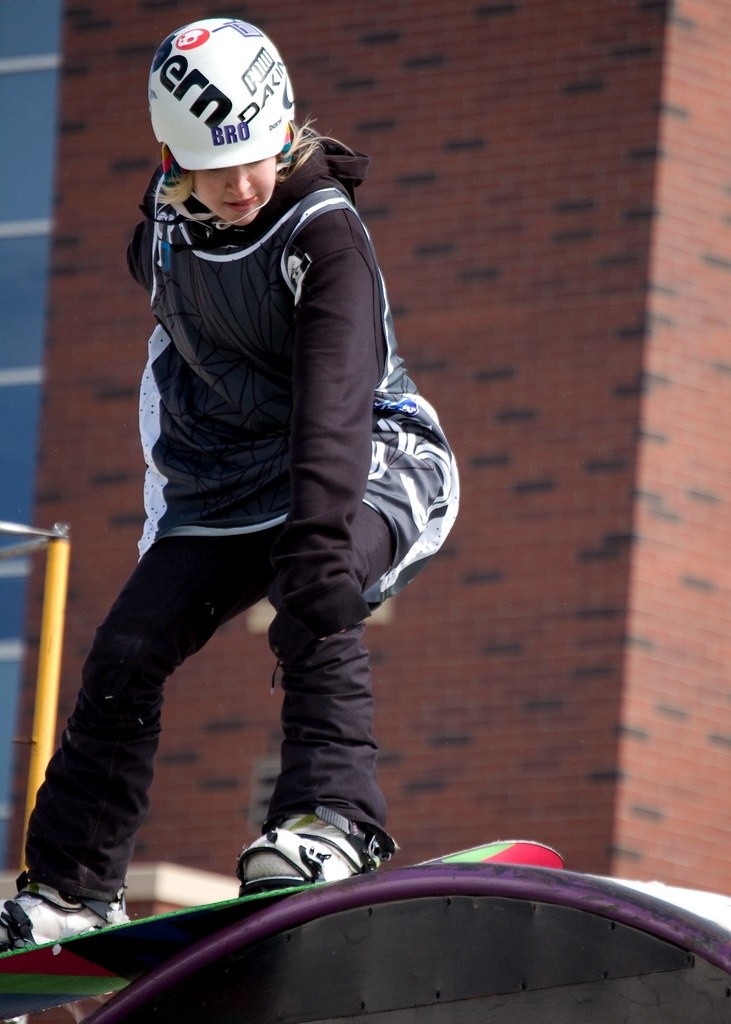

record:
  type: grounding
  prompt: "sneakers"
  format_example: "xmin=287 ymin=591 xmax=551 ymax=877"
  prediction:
xmin=244 ymin=806 xmax=385 ymax=879
xmin=0 ymin=879 xmax=130 ymax=951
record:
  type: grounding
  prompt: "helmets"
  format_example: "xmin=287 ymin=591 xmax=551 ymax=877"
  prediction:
xmin=147 ymin=17 xmax=295 ymax=172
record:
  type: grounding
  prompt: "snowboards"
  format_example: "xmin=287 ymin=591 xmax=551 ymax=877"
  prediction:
xmin=1 ymin=839 xmax=562 ymax=1019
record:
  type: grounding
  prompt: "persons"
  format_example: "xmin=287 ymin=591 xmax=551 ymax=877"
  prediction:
xmin=0 ymin=15 xmax=462 ymax=953
